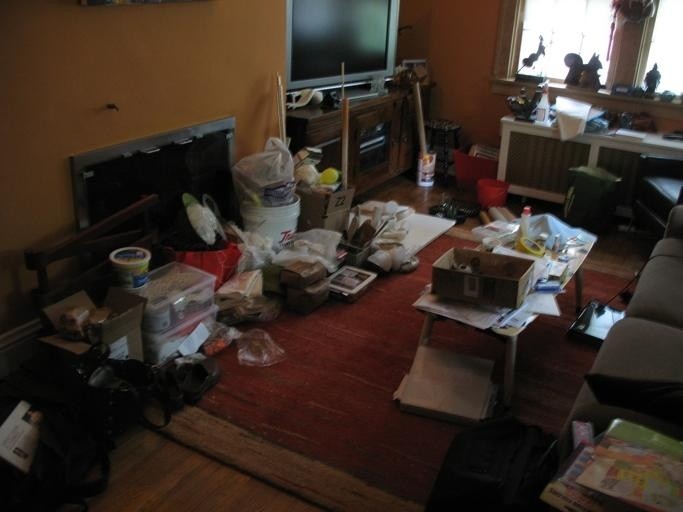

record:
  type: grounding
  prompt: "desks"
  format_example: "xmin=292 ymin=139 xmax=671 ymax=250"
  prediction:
xmin=412 ymin=212 xmax=598 ymax=407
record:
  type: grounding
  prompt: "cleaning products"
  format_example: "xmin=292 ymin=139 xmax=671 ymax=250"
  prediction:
xmin=535 ymin=80 xmax=550 ymax=126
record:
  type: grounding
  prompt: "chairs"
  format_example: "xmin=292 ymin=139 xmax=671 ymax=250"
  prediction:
xmin=630 ymin=131 xmax=682 ymax=227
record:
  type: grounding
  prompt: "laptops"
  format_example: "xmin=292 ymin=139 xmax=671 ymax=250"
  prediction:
xmin=568 ymin=299 xmax=625 ymax=344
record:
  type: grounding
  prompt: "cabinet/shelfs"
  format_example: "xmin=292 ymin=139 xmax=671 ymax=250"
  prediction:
xmin=404 ymin=82 xmax=438 ymax=173
xmin=497 ymin=110 xmax=683 ymax=220
xmin=288 ymin=84 xmax=409 ymax=198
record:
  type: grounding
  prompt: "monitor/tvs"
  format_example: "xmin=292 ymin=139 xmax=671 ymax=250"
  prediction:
xmin=286 ymin=0 xmax=402 ymax=90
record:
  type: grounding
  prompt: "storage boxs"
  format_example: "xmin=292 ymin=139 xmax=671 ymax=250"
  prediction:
xmin=295 ymin=186 xmax=356 ymax=235
xmin=117 ymin=261 xmax=218 ymax=336
xmin=140 ymin=304 xmax=220 ymax=366
xmin=431 ymin=245 xmax=534 ymax=309
xmin=38 ymin=286 xmax=150 ymax=364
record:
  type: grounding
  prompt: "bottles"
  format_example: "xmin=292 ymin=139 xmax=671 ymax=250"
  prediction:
xmin=536 ymin=87 xmax=549 ymax=123
xmin=521 ymin=207 xmax=531 ymax=238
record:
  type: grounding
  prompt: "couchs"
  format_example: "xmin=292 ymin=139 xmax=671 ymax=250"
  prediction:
xmin=558 ymin=206 xmax=682 ymax=464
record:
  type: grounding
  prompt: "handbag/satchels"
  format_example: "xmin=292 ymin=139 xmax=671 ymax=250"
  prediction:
xmin=93 ymin=358 xmax=153 ymax=441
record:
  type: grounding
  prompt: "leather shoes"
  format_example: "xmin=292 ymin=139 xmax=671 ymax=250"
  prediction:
xmin=169 ymin=357 xmax=220 ymax=409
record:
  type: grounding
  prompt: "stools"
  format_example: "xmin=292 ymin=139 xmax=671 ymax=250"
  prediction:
xmin=424 ymin=117 xmax=461 ymax=178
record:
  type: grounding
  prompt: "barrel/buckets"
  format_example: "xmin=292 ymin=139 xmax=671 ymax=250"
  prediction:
xmin=476 ymin=178 xmax=510 ymax=209
xmin=109 ymin=245 xmax=152 ymax=289
xmin=241 ymin=194 xmax=302 ymax=249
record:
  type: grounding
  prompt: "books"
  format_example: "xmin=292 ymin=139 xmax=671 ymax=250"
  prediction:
xmin=326 ymin=264 xmax=377 ymax=297
xmin=615 ymin=128 xmax=649 ymax=142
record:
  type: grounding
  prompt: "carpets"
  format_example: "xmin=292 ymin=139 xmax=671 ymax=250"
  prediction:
xmin=138 ymin=225 xmax=637 ymax=512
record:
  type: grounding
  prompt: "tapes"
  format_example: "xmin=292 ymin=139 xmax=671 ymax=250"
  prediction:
xmin=517 ymin=237 xmax=545 ymax=258
